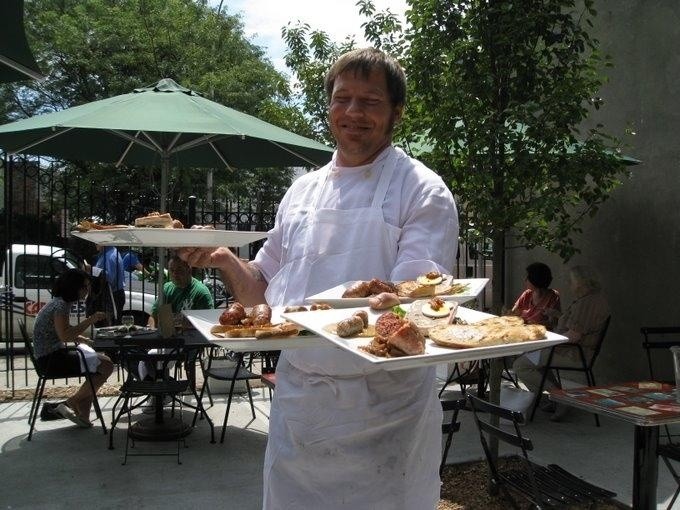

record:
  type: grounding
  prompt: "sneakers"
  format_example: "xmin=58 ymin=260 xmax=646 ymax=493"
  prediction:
xmin=541 ymin=401 xmax=571 ymax=420
xmin=143 ymin=393 xmax=174 ymax=414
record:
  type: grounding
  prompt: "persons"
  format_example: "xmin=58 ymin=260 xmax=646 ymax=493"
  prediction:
xmin=122 ymin=246 xmax=153 ymax=283
xmin=500 ymin=261 xmax=567 ymax=334
xmin=135 ymin=256 xmax=214 ymax=413
xmin=84 ymin=242 xmax=126 ymax=328
xmin=175 ymin=46 xmax=463 ymax=509
xmin=31 ymin=266 xmax=116 ymax=430
xmin=511 ymin=263 xmax=613 ymax=427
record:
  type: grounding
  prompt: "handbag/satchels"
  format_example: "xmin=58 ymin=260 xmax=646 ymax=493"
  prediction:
xmin=42 ymin=402 xmax=69 ymax=420
xmin=499 ymin=385 xmax=536 ymax=426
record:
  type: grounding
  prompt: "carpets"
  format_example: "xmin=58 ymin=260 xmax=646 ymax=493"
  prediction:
xmin=438 ymin=454 xmax=636 ymax=510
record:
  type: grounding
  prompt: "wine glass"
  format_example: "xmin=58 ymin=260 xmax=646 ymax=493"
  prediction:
xmin=121 ymin=316 xmax=134 ymax=335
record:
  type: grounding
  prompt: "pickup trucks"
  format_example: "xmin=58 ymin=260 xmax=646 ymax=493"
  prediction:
xmin=0 ymin=243 xmax=156 ymax=343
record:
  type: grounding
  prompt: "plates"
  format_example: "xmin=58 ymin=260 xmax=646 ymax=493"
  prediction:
xmin=179 ymin=304 xmax=334 ymax=352
xmin=303 ymin=276 xmax=489 ymax=308
xmin=278 ymin=301 xmax=572 ymax=373
xmin=94 ymin=325 xmax=160 ymax=338
xmin=70 ymin=226 xmax=275 ymax=248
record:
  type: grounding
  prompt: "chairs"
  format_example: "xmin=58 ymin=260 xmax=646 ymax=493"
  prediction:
xmin=17 ymin=309 xmax=263 ymax=470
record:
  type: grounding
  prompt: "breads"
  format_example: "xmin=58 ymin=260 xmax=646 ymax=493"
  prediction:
xmin=135 ymin=214 xmax=173 ymax=227
xmin=404 ymin=298 xmax=459 ymax=338
xmin=428 ymin=315 xmax=546 ymax=349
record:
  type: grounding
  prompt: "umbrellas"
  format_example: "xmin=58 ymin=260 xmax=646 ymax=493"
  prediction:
xmin=0 ymin=73 xmax=338 ymax=310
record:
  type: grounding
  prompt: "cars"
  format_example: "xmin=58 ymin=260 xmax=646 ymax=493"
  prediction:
xmin=469 ymin=237 xmax=494 ymax=260
xmin=131 ymin=255 xmax=227 ymax=309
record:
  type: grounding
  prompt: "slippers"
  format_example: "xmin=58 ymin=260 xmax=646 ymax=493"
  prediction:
xmin=57 ymin=404 xmax=92 ymax=427
